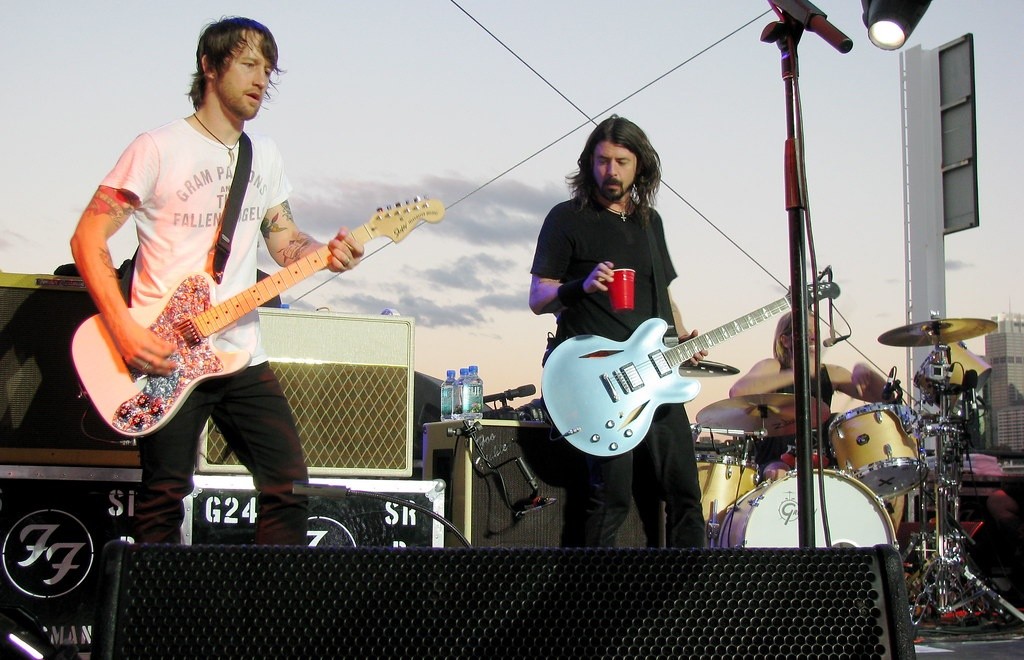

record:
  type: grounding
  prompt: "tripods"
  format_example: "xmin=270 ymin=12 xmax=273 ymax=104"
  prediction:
xmin=870 ymin=344 xmax=1024 ymax=625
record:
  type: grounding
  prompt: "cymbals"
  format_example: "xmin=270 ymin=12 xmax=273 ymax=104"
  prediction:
xmin=877 ymin=317 xmax=998 ymax=349
xmin=695 ymin=390 xmax=831 ymax=440
xmin=680 ymin=360 xmax=742 ymax=378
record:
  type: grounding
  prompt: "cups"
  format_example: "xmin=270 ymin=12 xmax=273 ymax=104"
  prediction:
xmin=607 ymin=269 xmax=635 ymax=313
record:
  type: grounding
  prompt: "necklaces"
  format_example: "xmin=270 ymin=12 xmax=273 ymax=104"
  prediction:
xmin=193 ymin=113 xmax=239 ymax=167
xmin=603 ymin=198 xmax=632 ymax=221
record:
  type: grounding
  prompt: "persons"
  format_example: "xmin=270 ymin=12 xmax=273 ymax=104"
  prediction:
xmin=529 ymin=114 xmax=708 ymax=547
xmin=70 ymin=18 xmax=364 ymax=544
xmin=729 ymin=311 xmax=896 ymax=483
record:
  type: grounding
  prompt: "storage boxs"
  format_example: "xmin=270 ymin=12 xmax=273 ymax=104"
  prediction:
xmin=1 ymin=466 xmax=446 ymax=660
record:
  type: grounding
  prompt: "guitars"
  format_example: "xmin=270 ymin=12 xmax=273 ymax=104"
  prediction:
xmin=542 ymin=276 xmax=840 ymax=457
xmin=69 ymin=193 xmax=445 ymax=436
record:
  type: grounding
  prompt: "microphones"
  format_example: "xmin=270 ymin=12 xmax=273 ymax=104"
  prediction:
xmin=880 ymin=365 xmax=900 ymax=401
xmin=823 ymin=335 xmax=848 ymax=348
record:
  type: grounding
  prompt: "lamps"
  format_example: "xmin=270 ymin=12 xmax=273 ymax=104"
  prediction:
xmin=860 ymin=0 xmax=934 ymax=50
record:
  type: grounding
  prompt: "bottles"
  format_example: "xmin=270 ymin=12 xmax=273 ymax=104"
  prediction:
xmin=441 ymin=370 xmax=457 ymax=422
xmin=451 ymin=368 xmax=469 ymax=420
xmin=462 ymin=366 xmax=483 ymax=419
xmin=281 ymin=304 xmax=289 ymax=308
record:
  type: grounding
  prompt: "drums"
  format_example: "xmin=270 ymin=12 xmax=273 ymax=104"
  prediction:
xmin=913 ymin=342 xmax=996 ymax=412
xmin=695 ymin=454 xmax=900 ymax=551
xmin=825 ymin=402 xmax=930 ymax=502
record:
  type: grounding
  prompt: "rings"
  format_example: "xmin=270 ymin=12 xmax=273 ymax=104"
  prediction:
xmin=140 ymin=363 xmax=151 ymax=374
xmin=344 ymin=258 xmax=350 ymax=266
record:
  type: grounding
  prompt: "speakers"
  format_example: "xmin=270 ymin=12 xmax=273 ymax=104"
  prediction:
xmin=195 ymin=307 xmax=414 ymax=478
xmin=88 ymin=536 xmax=919 ymax=660
xmin=5 ymin=468 xmax=183 ymax=651
xmin=422 ymin=421 xmax=667 ymax=548
xmin=0 ymin=271 xmax=141 ymax=467
xmin=182 ymin=480 xmax=447 ymax=548
